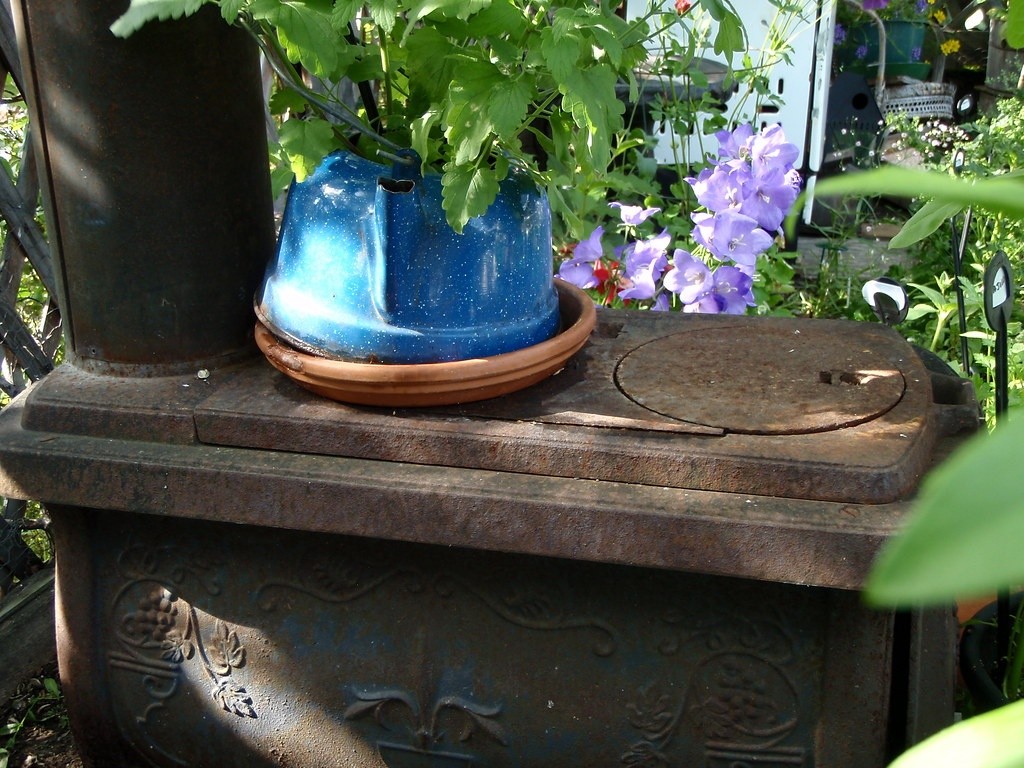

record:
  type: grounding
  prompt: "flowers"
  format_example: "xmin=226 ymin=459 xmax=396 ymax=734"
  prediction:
xmin=556 ymin=122 xmax=804 ymax=316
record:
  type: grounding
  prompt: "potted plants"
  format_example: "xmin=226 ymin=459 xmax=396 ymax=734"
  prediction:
xmin=110 ymin=1 xmax=750 ymax=397
xmin=837 ymin=1 xmax=932 ymax=82
